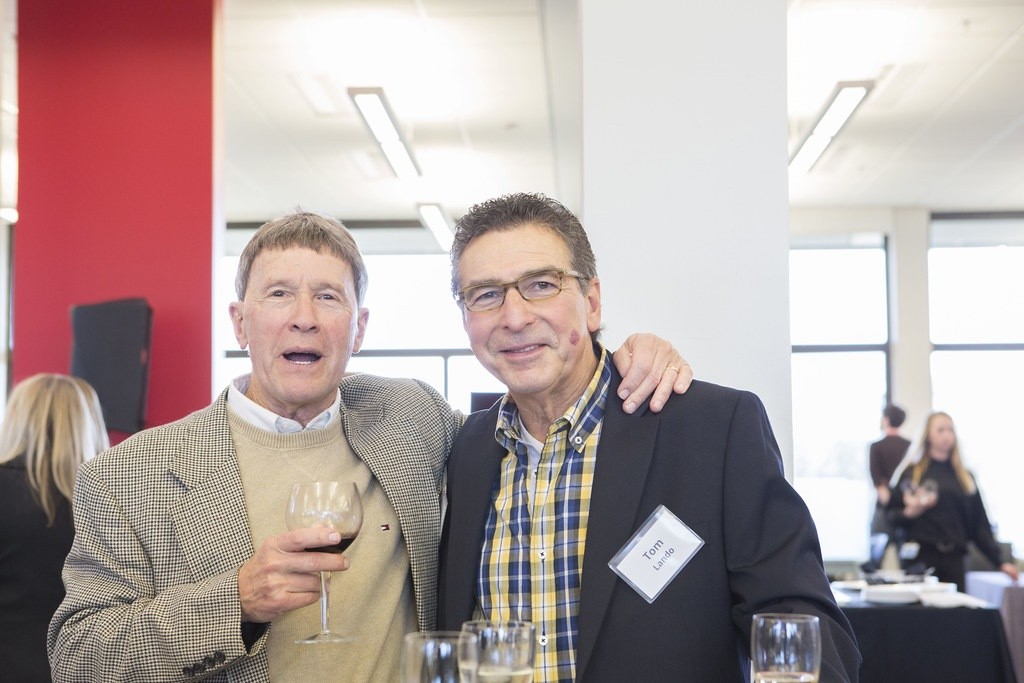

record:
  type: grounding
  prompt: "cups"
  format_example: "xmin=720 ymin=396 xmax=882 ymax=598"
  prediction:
xmin=457 ymin=619 xmax=537 ymax=683
xmin=750 ymin=613 xmax=822 ymax=683
xmin=400 ymin=631 xmax=478 ymax=683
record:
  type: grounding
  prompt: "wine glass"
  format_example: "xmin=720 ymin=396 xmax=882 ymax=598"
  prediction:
xmin=285 ymin=482 xmax=363 ymax=647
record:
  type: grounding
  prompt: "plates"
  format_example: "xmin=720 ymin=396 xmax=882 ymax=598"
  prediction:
xmin=861 ymin=584 xmax=919 ymax=604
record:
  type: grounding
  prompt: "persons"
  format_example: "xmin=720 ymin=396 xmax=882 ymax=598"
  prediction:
xmin=0 ymin=372 xmax=111 ymax=683
xmin=868 ymin=404 xmax=913 ymax=571
xmin=885 ymin=410 xmax=1018 ymax=593
xmin=432 ymin=197 xmax=864 ymax=683
xmin=46 ymin=211 xmax=693 ymax=683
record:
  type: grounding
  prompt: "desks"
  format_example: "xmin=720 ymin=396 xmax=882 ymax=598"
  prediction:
xmin=827 ymin=572 xmax=1024 ymax=683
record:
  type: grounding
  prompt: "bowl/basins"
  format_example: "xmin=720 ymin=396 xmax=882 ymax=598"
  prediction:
xmin=913 ymin=582 xmax=957 ymax=605
xmin=831 ymin=580 xmax=865 ymax=595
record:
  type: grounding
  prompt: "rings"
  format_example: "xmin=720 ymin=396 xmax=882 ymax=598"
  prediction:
xmin=667 ymin=365 xmax=680 ymax=374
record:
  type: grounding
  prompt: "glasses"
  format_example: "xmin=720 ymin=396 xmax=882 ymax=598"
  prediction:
xmin=454 ymin=269 xmax=590 ymax=313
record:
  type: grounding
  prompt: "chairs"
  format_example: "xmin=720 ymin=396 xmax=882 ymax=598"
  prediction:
xmin=962 ymin=540 xmax=1013 ymax=576
